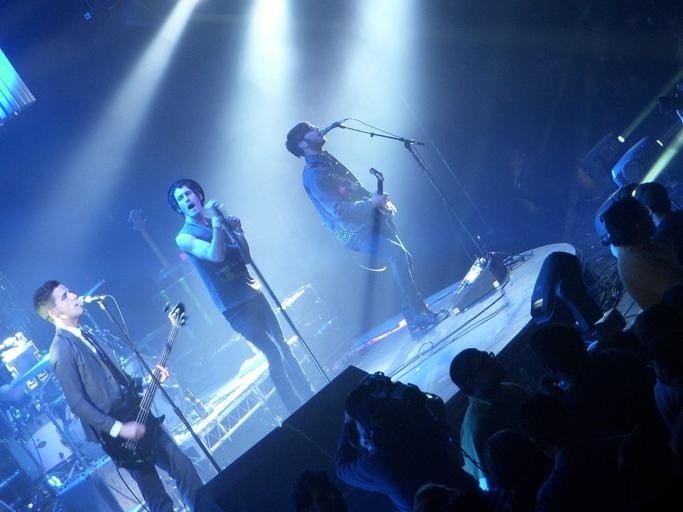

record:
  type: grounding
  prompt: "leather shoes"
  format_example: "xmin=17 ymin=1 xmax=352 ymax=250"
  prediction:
xmin=408 ymin=305 xmax=450 ymax=342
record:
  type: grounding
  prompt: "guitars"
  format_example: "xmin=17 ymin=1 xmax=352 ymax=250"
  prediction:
xmin=103 ymin=304 xmax=185 ymax=469
xmin=366 ymin=167 xmax=395 ymax=238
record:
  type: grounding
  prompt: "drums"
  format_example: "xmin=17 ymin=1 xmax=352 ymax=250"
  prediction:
xmin=64 ymin=416 xmax=103 ymax=458
xmin=124 ymin=354 xmax=153 ymax=381
xmin=27 ymin=418 xmax=73 ymax=470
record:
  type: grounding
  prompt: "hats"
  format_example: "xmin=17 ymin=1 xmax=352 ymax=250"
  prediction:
xmin=167 ymin=178 xmax=205 ymax=216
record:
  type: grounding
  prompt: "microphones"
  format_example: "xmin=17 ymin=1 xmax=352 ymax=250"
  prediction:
xmin=318 ymin=117 xmax=347 ymax=136
xmin=77 ymin=294 xmax=106 ymax=305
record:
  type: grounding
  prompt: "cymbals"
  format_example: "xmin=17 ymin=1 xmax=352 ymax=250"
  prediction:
xmin=77 ymin=279 xmax=108 ymax=298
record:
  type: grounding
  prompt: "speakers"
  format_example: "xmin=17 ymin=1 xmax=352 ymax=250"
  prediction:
xmin=57 ymin=472 xmax=126 ymax=512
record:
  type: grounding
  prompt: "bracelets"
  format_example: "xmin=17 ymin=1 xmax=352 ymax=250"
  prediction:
xmin=211 ymin=222 xmax=222 ymax=230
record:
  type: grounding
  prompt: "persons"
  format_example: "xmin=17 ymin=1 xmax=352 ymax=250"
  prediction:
xmin=604 ymin=197 xmax=680 ymax=309
xmin=286 ymin=120 xmax=449 ymax=341
xmin=35 ymin=280 xmax=203 ymax=512
xmin=450 ymin=350 xmax=531 ymax=492
xmin=336 ymin=386 xmax=482 ymax=509
xmin=636 ymin=183 xmax=680 ymax=246
xmin=521 ymin=393 xmax=674 ymax=509
xmin=635 ymin=303 xmax=681 ymax=439
xmin=531 ymin=324 xmax=670 ymax=445
xmin=167 ymin=178 xmax=315 ymax=416
xmin=484 ymin=429 xmax=554 ymax=509
